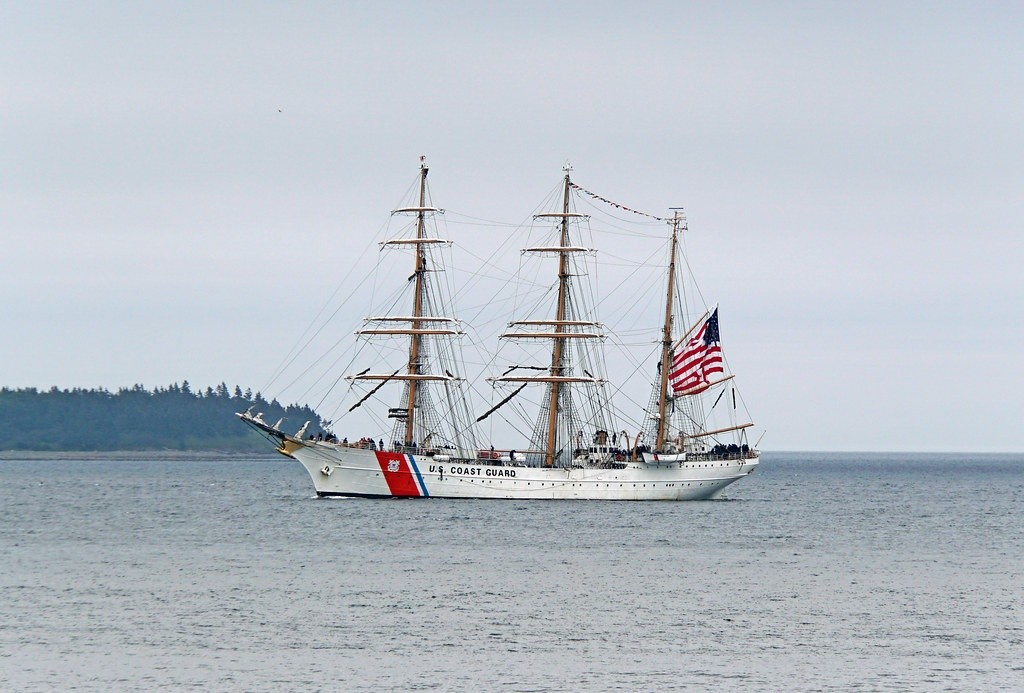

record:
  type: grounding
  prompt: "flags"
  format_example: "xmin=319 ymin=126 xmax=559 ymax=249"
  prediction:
xmin=654 ymin=453 xmax=658 ymax=460
xmin=669 ymin=304 xmax=723 ymax=398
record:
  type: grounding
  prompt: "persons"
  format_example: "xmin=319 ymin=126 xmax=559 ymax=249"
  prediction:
xmin=379 ymin=439 xmax=385 ymax=450
xmin=318 ymin=432 xmax=322 ymax=440
xmin=657 ymin=360 xmax=663 ymax=375
xmin=613 ymin=443 xmax=749 ymax=463
xmin=343 ymin=438 xmax=348 ymax=447
xmin=490 ymin=444 xmax=494 ymax=455
xmin=509 ymin=450 xmax=516 ymax=461
xmin=359 ymin=437 xmax=376 ymax=450
xmin=325 ymin=433 xmax=336 ymax=443
xmin=309 ymin=433 xmax=314 ymax=440
xmin=394 ymin=441 xmax=417 ymax=454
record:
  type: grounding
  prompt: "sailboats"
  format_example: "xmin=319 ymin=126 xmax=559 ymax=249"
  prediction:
xmin=233 ymin=151 xmax=760 ymax=504
